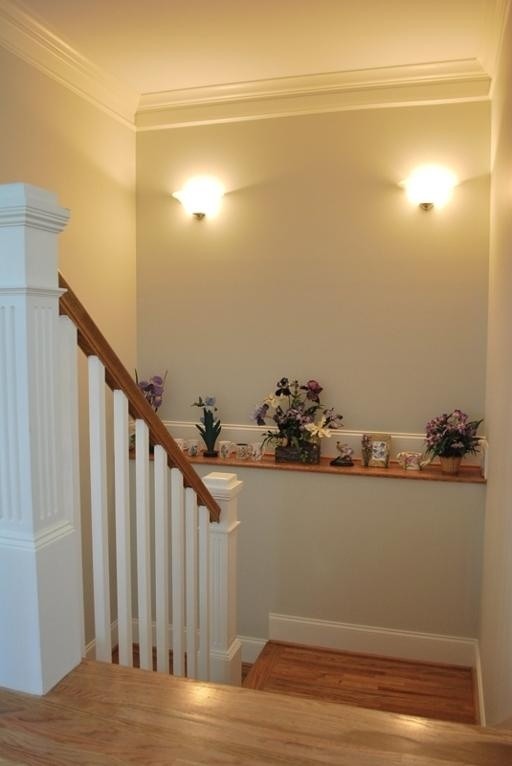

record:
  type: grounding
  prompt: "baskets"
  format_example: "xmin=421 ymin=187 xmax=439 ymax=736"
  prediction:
xmin=439 ymin=456 xmax=462 ymax=473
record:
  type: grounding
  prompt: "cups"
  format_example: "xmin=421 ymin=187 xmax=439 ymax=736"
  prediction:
xmin=175 ymin=439 xmax=204 ymax=457
xmin=218 ymin=441 xmax=265 ymax=461
xmin=397 ymin=452 xmax=422 ymax=470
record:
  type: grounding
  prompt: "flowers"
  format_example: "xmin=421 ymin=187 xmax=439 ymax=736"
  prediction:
xmin=189 ymin=394 xmax=222 ymax=432
xmin=422 ymin=409 xmax=489 ymax=464
xmin=248 ymin=377 xmax=344 ymax=464
xmin=133 ymin=369 xmax=167 ymax=414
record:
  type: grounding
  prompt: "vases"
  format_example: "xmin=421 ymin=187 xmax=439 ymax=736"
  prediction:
xmin=440 ymin=455 xmax=461 ymax=474
xmin=200 ymin=433 xmax=219 ymax=457
xmin=274 ymin=445 xmax=320 ymax=464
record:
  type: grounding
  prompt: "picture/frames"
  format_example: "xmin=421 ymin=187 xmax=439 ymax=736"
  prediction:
xmin=367 ymin=434 xmax=391 ymax=467
